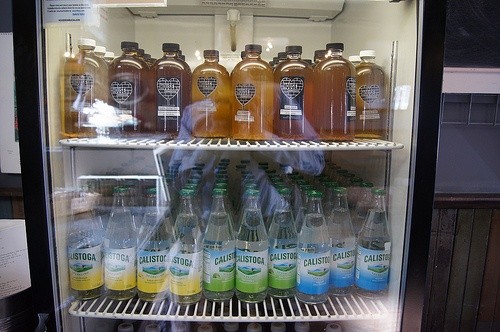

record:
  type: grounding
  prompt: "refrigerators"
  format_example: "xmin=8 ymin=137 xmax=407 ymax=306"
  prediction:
xmin=12 ymin=0 xmax=442 ymax=332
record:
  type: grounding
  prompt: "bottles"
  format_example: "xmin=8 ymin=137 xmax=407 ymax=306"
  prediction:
xmin=109 ymin=307 xmax=343 ymax=332
xmin=62 ymin=156 xmax=393 ymax=304
xmin=60 ymin=37 xmax=390 ymax=143
